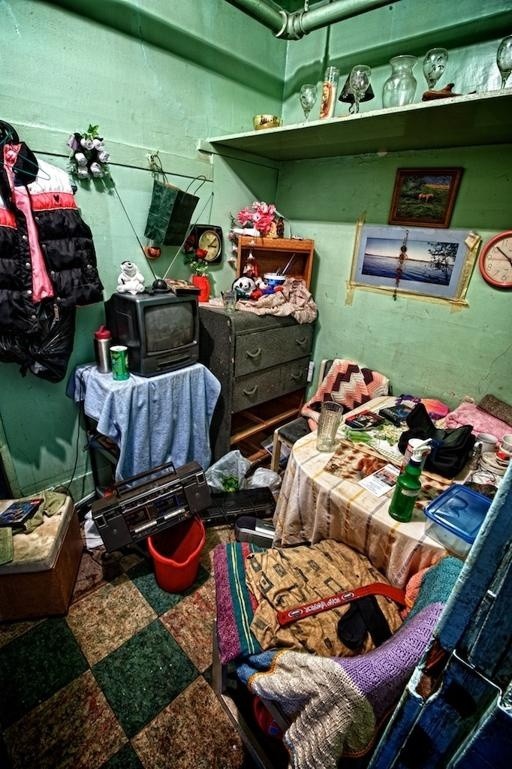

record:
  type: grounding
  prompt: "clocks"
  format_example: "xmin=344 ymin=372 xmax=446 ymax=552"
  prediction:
xmin=479 ymin=231 xmax=512 ymax=290
xmin=189 ymin=224 xmax=224 ymax=266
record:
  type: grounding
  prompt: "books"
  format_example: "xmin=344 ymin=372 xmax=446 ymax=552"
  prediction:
xmin=379 ymin=402 xmax=416 ymax=424
xmin=164 ymin=279 xmax=201 ymax=296
xmin=0 ymin=499 xmax=42 ymax=527
xmin=346 ymin=402 xmax=385 ymax=429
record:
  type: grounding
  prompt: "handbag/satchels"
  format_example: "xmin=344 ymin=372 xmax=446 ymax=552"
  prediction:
xmin=143 ymin=156 xmax=206 ymax=246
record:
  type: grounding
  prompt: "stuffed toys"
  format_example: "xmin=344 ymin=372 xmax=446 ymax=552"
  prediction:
xmin=232 ymin=276 xmax=257 ymax=299
xmin=116 ymin=261 xmax=146 ymax=294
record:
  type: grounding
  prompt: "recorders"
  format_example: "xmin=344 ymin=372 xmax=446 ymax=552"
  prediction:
xmin=232 ymin=517 xmax=275 ymax=548
xmin=92 ymin=460 xmax=213 ymax=553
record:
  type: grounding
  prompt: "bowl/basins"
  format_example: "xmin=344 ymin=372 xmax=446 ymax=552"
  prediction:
xmin=252 ymin=114 xmax=282 ymax=131
xmin=480 ymin=435 xmax=512 ymax=476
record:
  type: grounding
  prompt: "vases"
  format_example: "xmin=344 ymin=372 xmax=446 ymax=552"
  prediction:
xmin=190 ymin=276 xmax=211 ymax=302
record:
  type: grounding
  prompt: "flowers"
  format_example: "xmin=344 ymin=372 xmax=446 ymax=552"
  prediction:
xmin=228 ymin=201 xmax=277 ymax=237
xmin=182 ymin=236 xmax=208 ymax=278
xmin=66 ymin=123 xmax=110 ymax=181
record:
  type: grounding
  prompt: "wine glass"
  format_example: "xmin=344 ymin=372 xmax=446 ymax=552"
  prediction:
xmin=299 ymin=84 xmax=317 ymax=123
xmin=493 ymin=36 xmax=512 ymax=91
xmin=349 ymin=66 xmax=371 ymax=113
xmin=424 ymin=46 xmax=447 ymax=101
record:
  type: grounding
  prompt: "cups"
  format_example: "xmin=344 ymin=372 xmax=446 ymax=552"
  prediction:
xmin=222 ymin=290 xmax=236 ymax=313
xmin=261 ymin=272 xmax=287 ymax=287
xmin=110 ymin=346 xmax=129 ymax=380
xmin=474 ymin=431 xmax=497 ymax=458
xmin=316 ymin=401 xmax=344 ymax=450
xmin=400 ymin=437 xmax=430 ymax=478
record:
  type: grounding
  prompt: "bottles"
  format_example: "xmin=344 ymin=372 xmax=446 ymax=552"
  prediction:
xmin=379 ymin=53 xmax=417 ymax=107
xmin=95 ymin=325 xmax=114 ymax=373
xmin=277 ymin=216 xmax=284 ymax=237
xmin=382 ymin=443 xmax=427 ymax=526
xmin=320 ymin=66 xmax=337 ymax=119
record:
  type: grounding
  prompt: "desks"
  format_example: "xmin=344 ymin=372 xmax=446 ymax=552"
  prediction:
xmin=67 ymin=362 xmax=221 ymax=496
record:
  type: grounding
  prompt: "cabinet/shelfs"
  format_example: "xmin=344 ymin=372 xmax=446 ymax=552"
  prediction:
xmin=196 ymin=300 xmax=317 ymax=476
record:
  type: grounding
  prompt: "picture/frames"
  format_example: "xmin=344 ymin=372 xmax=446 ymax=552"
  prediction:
xmin=387 ymin=165 xmax=466 ymax=229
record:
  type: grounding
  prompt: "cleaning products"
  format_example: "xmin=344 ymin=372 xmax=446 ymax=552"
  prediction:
xmin=388 ymin=437 xmax=434 ymax=523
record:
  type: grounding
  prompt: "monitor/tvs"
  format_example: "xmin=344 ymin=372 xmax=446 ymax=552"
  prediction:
xmin=104 ymin=289 xmax=200 ymax=378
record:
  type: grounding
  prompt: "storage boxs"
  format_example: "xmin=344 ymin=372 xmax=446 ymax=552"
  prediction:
xmin=0 ymin=495 xmax=86 ymax=621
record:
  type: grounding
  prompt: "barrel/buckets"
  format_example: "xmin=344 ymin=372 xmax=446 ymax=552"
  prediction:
xmin=145 ymin=518 xmax=207 ymax=594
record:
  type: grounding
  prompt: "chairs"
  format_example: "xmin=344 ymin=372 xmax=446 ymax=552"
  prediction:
xmin=270 ymin=355 xmax=393 ymax=475
xmin=212 ymin=540 xmax=468 ymax=769
